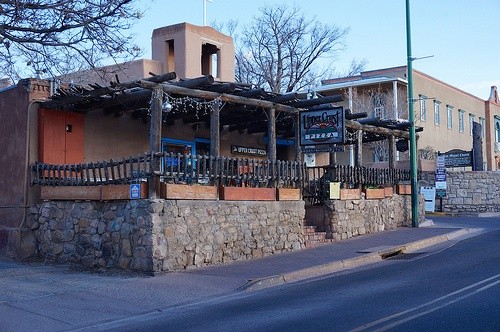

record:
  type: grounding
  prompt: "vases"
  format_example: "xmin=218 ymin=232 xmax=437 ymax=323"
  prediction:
xmin=39 ymin=173 xmax=414 ymax=202
